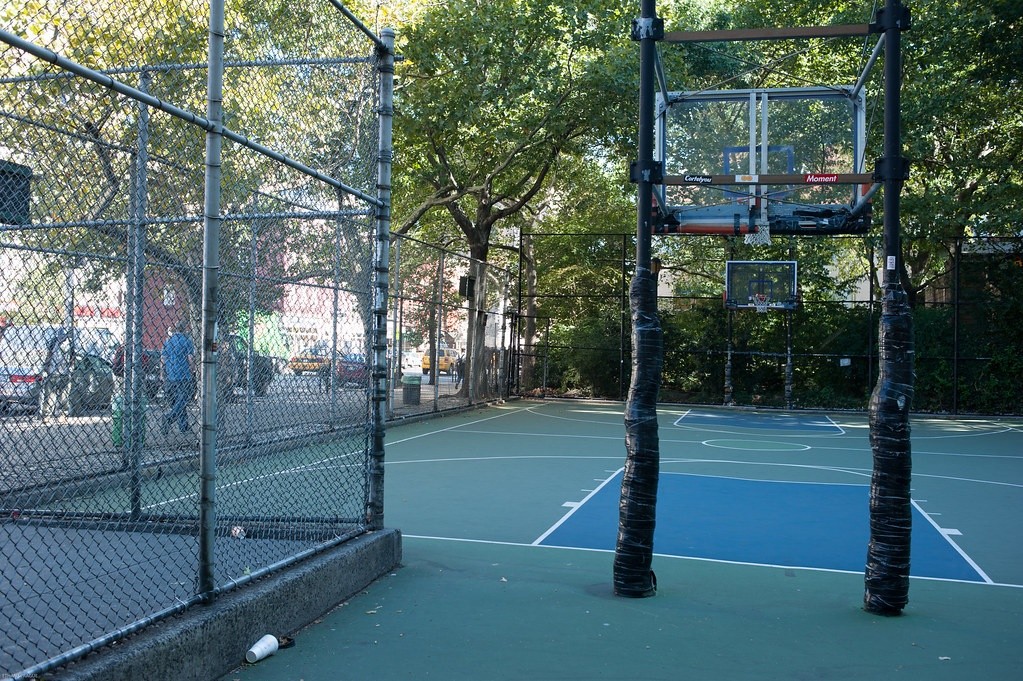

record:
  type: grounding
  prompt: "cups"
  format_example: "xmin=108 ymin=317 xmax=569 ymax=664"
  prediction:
xmin=246 ymin=634 xmax=278 ymax=663
xmin=232 ymin=527 xmax=245 ymax=538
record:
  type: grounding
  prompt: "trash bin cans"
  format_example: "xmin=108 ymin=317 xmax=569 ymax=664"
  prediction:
xmin=110 ymin=392 xmax=146 ymax=450
xmin=401 ymin=374 xmax=421 ymax=405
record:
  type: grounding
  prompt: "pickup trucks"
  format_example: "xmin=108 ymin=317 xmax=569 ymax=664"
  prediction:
xmin=113 ymin=333 xmax=273 ymax=400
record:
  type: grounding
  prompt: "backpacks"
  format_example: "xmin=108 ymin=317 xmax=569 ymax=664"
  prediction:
xmin=456 ymin=354 xmax=465 ymax=369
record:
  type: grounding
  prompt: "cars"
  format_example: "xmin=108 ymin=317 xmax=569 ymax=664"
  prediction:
xmin=291 ymin=348 xmax=368 ymax=388
xmin=0 ymin=354 xmax=114 ymax=415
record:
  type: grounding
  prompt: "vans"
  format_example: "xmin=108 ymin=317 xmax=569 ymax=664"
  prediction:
xmin=422 ymin=348 xmax=462 ymax=374
xmin=1 ymin=324 xmax=117 ymax=364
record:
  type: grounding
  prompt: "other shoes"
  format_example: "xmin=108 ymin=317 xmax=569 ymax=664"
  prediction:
xmin=160 ymin=414 xmax=170 ymax=435
xmin=455 ymin=383 xmax=458 ymax=389
xmin=179 ymin=426 xmax=194 ymax=434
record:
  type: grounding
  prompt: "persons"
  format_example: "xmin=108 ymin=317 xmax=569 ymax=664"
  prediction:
xmin=318 ymin=360 xmax=331 ymax=396
xmin=160 ymin=319 xmax=197 ymax=436
xmin=0 ymin=315 xmax=14 ymax=339
xmin=454 ymin=348 xmax=466 ymax=389
xmin=38 ymin=322 xmax=74 ymax=424
xmin=111 ymin=331 xmax=150 ymax=448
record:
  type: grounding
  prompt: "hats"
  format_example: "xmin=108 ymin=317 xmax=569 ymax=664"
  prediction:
xmin=461 ymin=347 xmax=465 ymax=350
